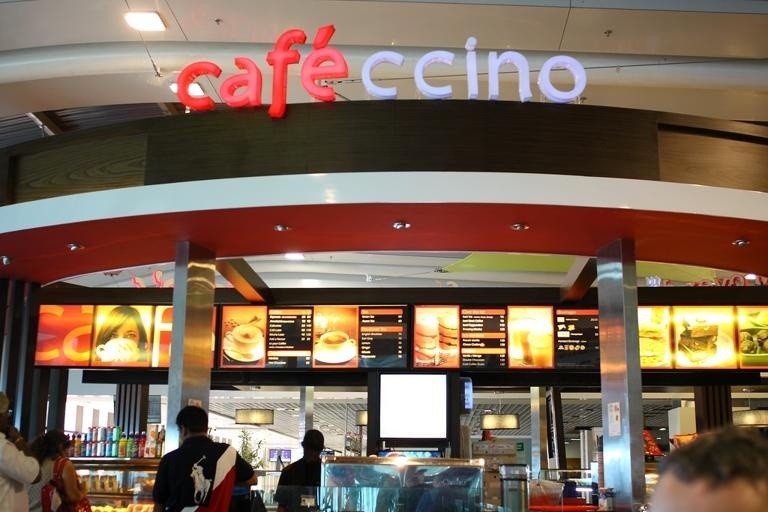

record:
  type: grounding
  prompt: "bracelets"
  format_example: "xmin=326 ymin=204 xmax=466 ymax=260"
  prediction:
xmin=14 ymin=437 xmax=24 ymax=445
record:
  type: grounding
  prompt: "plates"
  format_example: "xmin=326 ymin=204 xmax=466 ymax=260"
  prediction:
xmin=314 ymin=344 xmax=356 ymax=363
xmin=221 ymin=335 xmax=265 ymax=363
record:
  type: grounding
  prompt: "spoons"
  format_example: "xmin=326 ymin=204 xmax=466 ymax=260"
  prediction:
xmin=223 ymin=349 xmax=253 ymax=359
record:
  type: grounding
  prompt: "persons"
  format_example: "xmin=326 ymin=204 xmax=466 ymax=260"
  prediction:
xmin=0 ymin=390 xmax=40 ymax=512
xmin=274 ymin=430 xmax=324 ymax=512
xmin=650 ymin=424 xmax=768 ymax=512
xmin=95 ymin=307 xmax=148 ymax=352
xmin=152 ymin=405 xmax=258 ymax=512
xmin=27 ymin=429 xmax=87 ymax=512
xmin=563 ymin=481 xmax=578 ymax=497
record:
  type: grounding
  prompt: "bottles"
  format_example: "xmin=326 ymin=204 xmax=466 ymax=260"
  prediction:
xmin=68 ymin=426 xmax=146 ymax=458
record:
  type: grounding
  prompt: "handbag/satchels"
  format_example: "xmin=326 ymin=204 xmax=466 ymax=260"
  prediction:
xmin=42 ymin=480 xmax=92 ymax=512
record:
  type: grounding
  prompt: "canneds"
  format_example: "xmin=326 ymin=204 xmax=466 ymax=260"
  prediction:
xmin=80 ymin=426 xmax=121 ymax=457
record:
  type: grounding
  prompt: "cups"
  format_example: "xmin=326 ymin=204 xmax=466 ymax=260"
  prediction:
xmin=225 ymin=323 xmax=262 ymax=352
xmin=519 ymin=330 xmax=552 ymax=367
xmin=319 ymin=331 xmax=356 ymax=352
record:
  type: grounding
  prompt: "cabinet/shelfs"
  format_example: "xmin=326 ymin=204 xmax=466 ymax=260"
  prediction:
xmin=66 ymin=457 xmax=161 ymax=511
